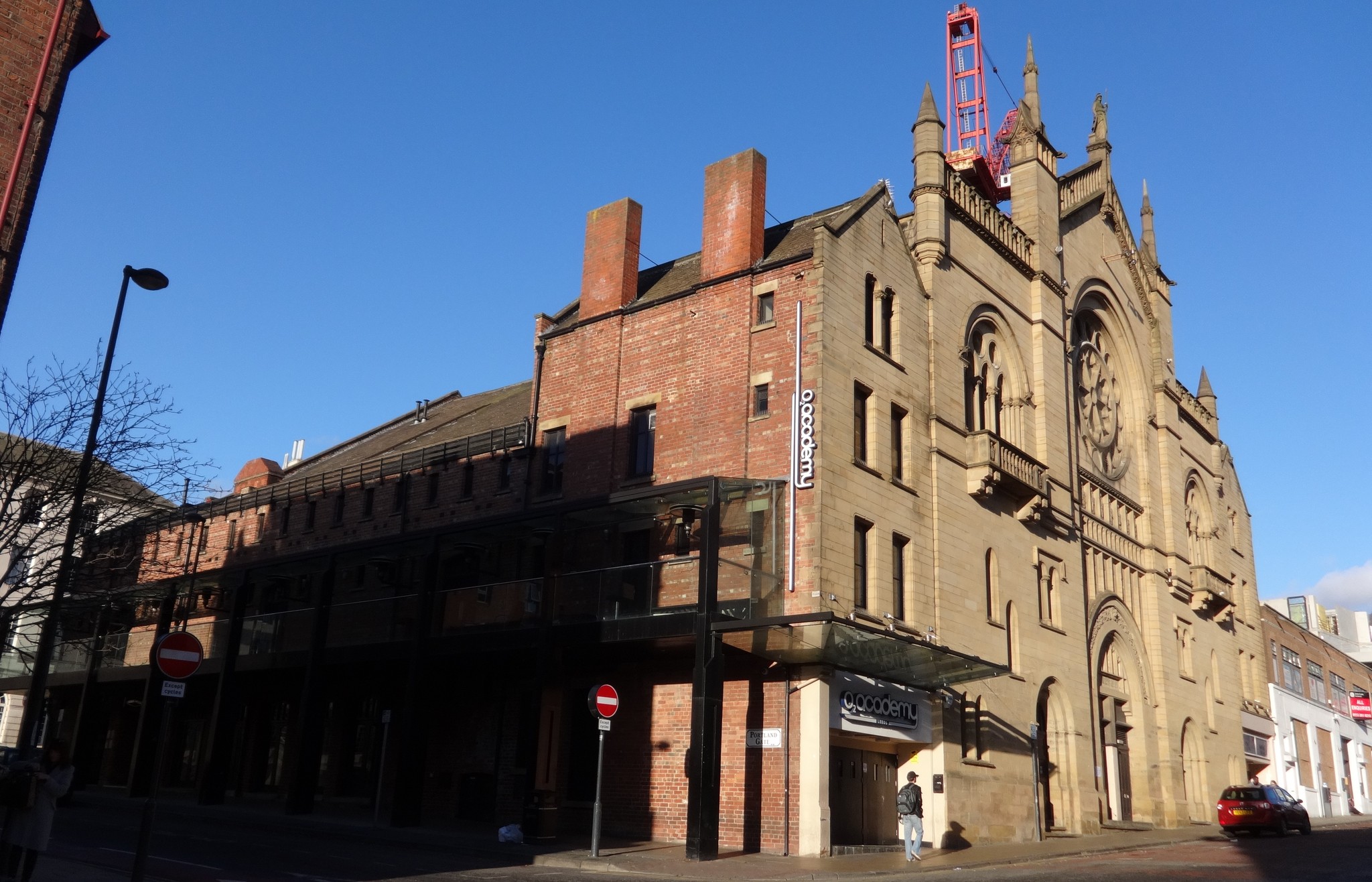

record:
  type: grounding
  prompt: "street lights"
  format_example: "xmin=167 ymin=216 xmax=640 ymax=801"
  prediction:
xmin=17 ymin=263 xmax=169 ymax=756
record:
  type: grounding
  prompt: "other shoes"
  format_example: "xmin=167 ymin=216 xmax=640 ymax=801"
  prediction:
xmin=908 ymin=857 xmax=916 ymax=862
xmin=911 ymin=850 xmax=921 ymax=861
xmin=8 ymin=877 xmax=16 ymax=882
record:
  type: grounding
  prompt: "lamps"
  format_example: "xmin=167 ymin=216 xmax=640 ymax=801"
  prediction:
xmin=920 ymin=634 xmax=932 ymax=642
xmin=884 ymin=623 xmax=896 ymax=633
xmin=844 ymin=611 xmax=857 ymax=622
xmin=669 ymin=503 xmax=706 ymax=541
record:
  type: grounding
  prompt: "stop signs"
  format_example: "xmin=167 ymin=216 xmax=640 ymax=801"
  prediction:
xmin=156 ymin=632 xmax=203 ymax=679
xmin=595 ymin=686 xmax=619 ymax=717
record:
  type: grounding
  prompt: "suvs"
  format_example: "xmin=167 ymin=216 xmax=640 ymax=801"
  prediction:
xmin=1216 ymin=783 xmax=1311 ymax=839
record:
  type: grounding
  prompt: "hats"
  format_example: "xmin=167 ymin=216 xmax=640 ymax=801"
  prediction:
xmin=907 ymin=771 xmax=919 ymax=778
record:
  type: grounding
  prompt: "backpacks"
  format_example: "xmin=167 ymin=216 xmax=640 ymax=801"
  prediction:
xmin=897 ymin=784 xmax=916 ymax=815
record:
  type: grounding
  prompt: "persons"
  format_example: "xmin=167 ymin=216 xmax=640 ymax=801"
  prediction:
xmin=1247 ymin=775 xmax=1262 ymax=787
xmin=0 ymin=736 xmax=75 ymax=882
xmin=1270 ymin=780 xmax=1277 ymax=785
xmin=898 ymin=771 xmax=924 ymax=862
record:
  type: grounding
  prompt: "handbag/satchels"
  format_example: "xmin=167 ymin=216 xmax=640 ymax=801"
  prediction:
xmin=7 ymin=768 xmax=40 ymax=809
